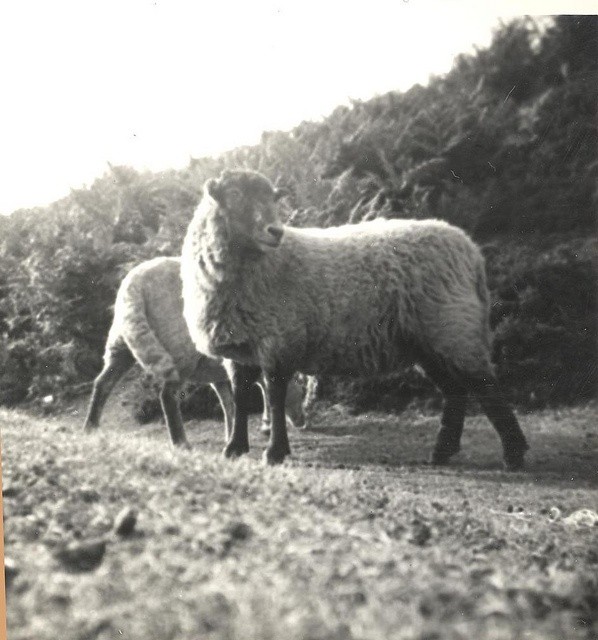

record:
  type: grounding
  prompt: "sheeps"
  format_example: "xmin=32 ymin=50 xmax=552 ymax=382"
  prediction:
xmin=178 ymin=168 xmax=529 ymax=474
xmin=82 ymin=256 xmax=310 ymax=452
